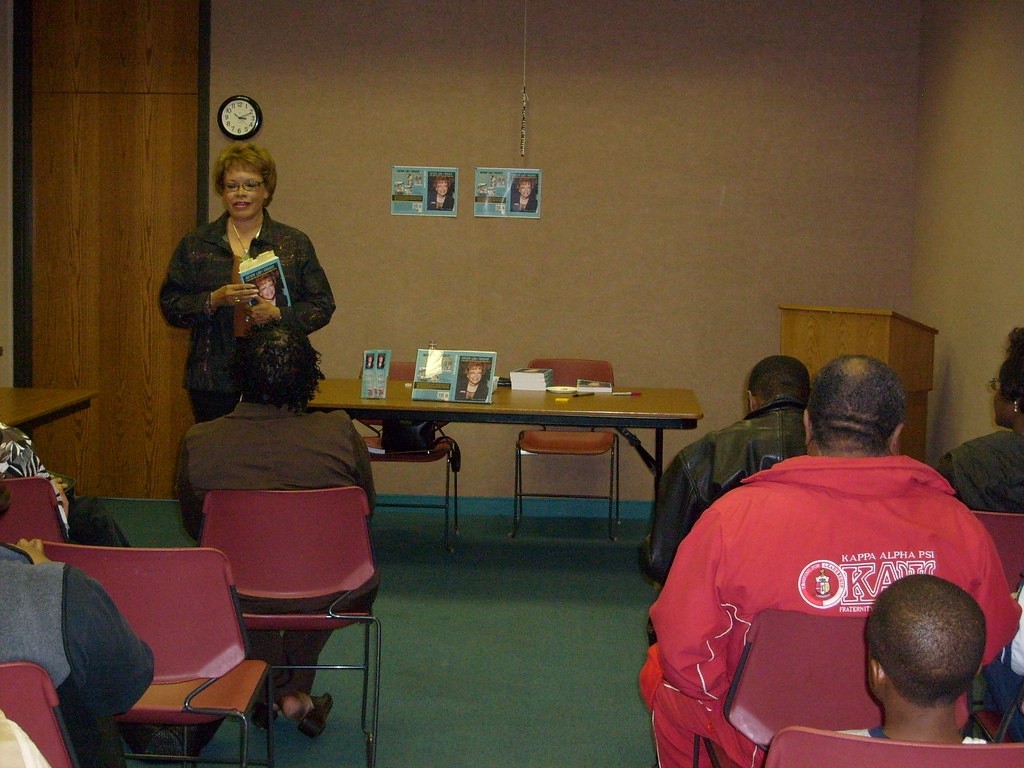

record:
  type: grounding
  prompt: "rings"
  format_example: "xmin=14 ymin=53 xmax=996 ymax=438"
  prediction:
xmin=245 ymin=316 xmax=250 ymax=322
xmin=234 ymin=297 xmax=240 ymax=303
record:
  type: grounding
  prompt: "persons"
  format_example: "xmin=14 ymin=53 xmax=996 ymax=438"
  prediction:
xmin=510 ymin=176 xmax=538 ymax=213
xmin=455 ymin=360 xmax=490 ymax=402
xmin=427 ymin=175 xmax=455 ymax=211
xmin=377 ymin=354 xmax=385 ymax=369
xmin=366 ymin=354 xmax=374 ymax=369
xmin=0 ymin=421 xmax=70 ymax=542
xmin=175 ymin=320 xmax=377 ymax=736
xmin=635 ymin=325 xmax=1024 ymax=768
xmin=0 ymin=483 xmax=184 ymax=768
xmin=252 ymin=272 xmax=288 ymax=307
xmin=160 ymin=143 xmax=336 ymax=422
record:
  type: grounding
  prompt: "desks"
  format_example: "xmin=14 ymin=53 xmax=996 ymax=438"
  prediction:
xmin=0 ymin=387 xmax=100 ymax=434
xmin=303 ymin=377 xmax=703 ymax=506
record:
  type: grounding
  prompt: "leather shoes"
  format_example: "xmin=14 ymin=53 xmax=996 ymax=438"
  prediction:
xmin=250 ymin=702 xmax=278 ymax=729
xmin=297 ymin=692 xmax=333 ymax=739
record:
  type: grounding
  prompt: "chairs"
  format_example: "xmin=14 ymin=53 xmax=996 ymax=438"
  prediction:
xmin=972 ymin=511 xmax=1024 ymax=598
xmin=0 ymin=476 xmax=382 ymax=768
xmin=353 ymin=363 xmax=460 ymax=554
xmin=764 ymin=725 xmax=1024 ymax=768
xmin=722 ymin=609 xmax=969 ymax=752
xmin=510 ymin=358 xmax=619 ymax=540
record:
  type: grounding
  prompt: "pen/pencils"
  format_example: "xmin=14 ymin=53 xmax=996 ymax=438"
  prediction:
xmin=612 ymin=392 xmax=642 ymax=396
xmin=573 ymin=392 xmax=594 ymax=397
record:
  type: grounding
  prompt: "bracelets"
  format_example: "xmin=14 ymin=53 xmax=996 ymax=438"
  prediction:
xmin=209 ymin=291 xmax=214 ymax=312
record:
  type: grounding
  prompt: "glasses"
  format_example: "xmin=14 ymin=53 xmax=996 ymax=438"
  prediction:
xmin=989 ymin=378 xmax=1002 ymax=392
xmin=221 ymin=180 xmax=265 ymax=193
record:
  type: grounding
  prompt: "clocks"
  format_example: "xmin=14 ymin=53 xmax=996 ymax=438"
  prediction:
xmin=217 ymin=95 xmax=262 ymax=141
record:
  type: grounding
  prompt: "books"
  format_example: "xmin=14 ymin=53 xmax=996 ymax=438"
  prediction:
xmin=577 ymin=379 xmax=612 ymax=393
xmin=510 ymin=368 xmax=553 ymax=392
xmin=238 ymin=257 xmax=291 ymax=308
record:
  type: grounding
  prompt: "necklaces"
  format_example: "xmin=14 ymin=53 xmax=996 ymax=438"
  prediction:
xmin=231 ymin=221 xmax=262 ymax=260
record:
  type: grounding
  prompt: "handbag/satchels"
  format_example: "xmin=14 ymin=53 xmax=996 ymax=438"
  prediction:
xmin=380 ymin=417 xmax=461 ymax=473
